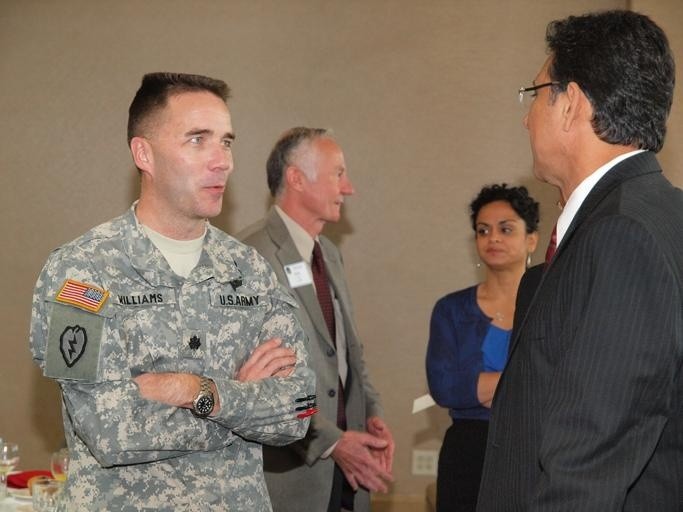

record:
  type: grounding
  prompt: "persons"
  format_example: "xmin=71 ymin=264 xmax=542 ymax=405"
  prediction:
xmin=238 ymin=127 xmax=394 ymax=512
xmin=30 ymin=72 xmax=317 ymax=512
xmin=425 ymin=181 xmax=541 ymax=512
xmin=478 ymin=11 xmax=682 ymax=512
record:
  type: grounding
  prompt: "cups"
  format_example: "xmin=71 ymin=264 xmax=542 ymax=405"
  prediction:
xmin=50 ymin=448 xmax=69 ymax=482
xmin=32 ymin=479 xmax=64 ymax=512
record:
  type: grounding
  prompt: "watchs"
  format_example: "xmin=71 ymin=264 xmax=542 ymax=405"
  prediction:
xmin=192 ymin=377 xmax=214 ymax=416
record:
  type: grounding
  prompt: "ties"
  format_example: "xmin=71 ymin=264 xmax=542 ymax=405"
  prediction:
xmin=311 ymin=240 xmax=338 ymax=353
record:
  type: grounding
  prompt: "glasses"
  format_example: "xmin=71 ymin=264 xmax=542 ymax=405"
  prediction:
xmin=517 ymin=80 xmax=561 ymax=115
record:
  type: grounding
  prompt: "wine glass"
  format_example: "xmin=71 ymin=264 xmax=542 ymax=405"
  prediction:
xmin=0 ymin=440 xmax=20 ymax=499
